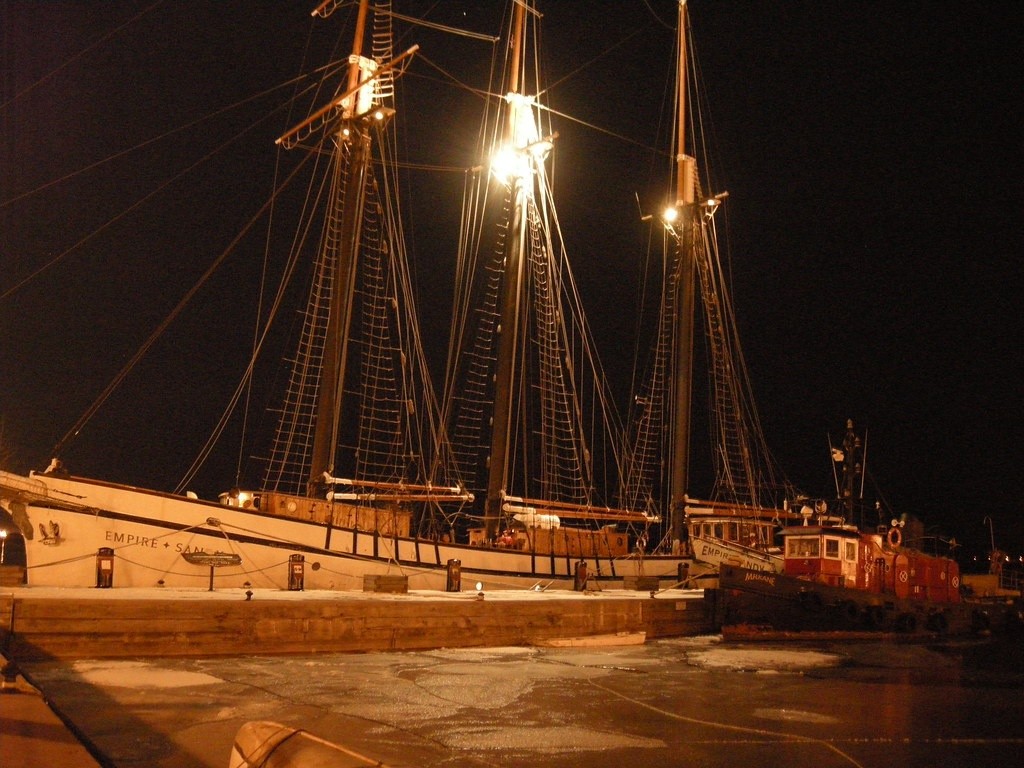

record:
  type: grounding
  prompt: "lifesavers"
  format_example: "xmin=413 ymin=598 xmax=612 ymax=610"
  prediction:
xmin=888 ymin=528 xmax=902 ymax=549
xmin=636 ymin=537 xmax=647 ymax=550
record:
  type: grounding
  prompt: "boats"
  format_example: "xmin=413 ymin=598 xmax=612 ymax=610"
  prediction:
xmin=689 ymin=418 xmax=1024 ymax=642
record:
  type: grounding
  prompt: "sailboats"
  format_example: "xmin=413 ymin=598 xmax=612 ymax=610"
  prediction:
xmin=0 ymin=0 xmax=786 ymax=588
